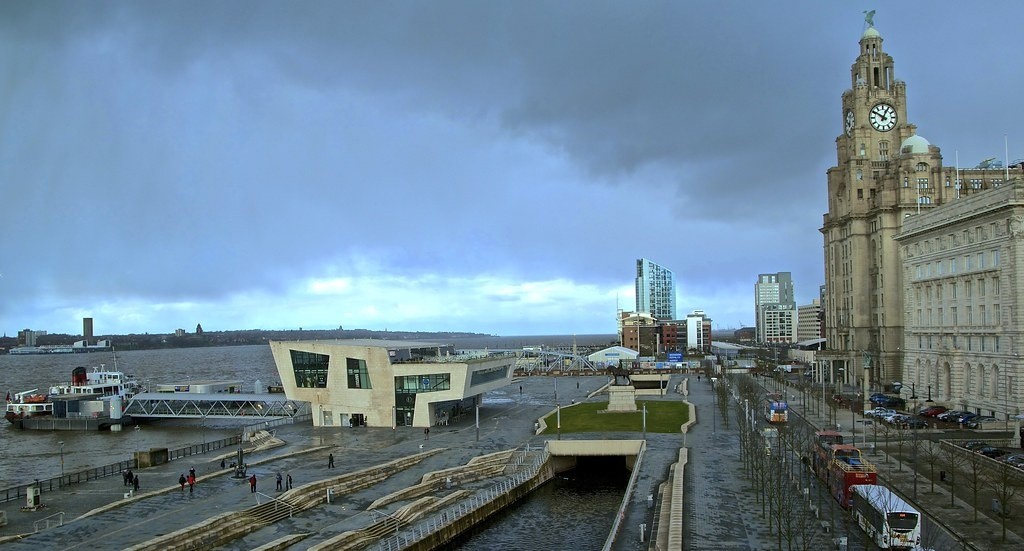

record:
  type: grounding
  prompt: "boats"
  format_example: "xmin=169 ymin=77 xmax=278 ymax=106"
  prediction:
xmin=5 ymin=346 xmax=147 ymax=421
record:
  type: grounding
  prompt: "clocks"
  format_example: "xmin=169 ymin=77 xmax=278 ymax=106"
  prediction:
xmin=868 ymin=102 xmax=898 ymax=133
xmin=845 ymin=111 xmax=855 ymax=138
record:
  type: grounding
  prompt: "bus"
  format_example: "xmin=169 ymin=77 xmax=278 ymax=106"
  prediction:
xmin=849 ymin=484 xmax=922 ymax=551
xmin=814 ymin=430 xmax=877 ymax=510
xmin=763 ymin=399 xmax=789 ymax=423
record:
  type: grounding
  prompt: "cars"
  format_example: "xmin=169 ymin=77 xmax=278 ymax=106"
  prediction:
xmin=919 ymin=405 xmax=999 ymax=428
xmin=773 ymin=368 xmax=787 ymax=373
xmin=804 ymin=370 xmax=816 ymax=377
xmin=865 ymin=393 xmax=928 ymax=430
xmin=964 ymin=441 xmax=1024 ymax=471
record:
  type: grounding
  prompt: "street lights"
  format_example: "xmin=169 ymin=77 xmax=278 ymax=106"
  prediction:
xmin=892 ymin=382 xmax=917 ymax=499
xmin=711 ymin=378 xmax=718 ymax=432
xmin=134 ymin=425 xmax=141 ymax=470
xmin=58 ymin=442 xmax=66 ymax=490
xmin=201 ymin=416 xmax=206 ymax=454
xmin=839 ymin=368 xmax=855 ymax=446
xmin=812 ymin=360 xmax=825 ymax=426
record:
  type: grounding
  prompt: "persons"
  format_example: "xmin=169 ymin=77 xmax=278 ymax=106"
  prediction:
xmin=249 ymin=474 xmax=257 ymax=493
xmin=285 ymin=474 xmax=292 ymax=491
xmin=618 ymin=360 xmax=623 ymax=376
xmin=122 ymin=469 xmax=139 ymax=491
xmin=436 ymin=410 xmax=449 ymax=426
xmin=276 ymin=473 xmax=282 ymax=491
xmin=424 ymin=427 xmax=430 ymax=440
xmin=349 ymin=418 xmax=353 ymax=428
xmin=698 ymin=376 xmax=700 ymax=381
xmin=801 ymin=453 xmax=811 ymax=473
xmin=179 ymin=465 xmax=197 ymax=492
xmin=328 ymin=453 xmax=335 ymax=469
xmin=221 ymin=458 xmax=236 ymax=470
